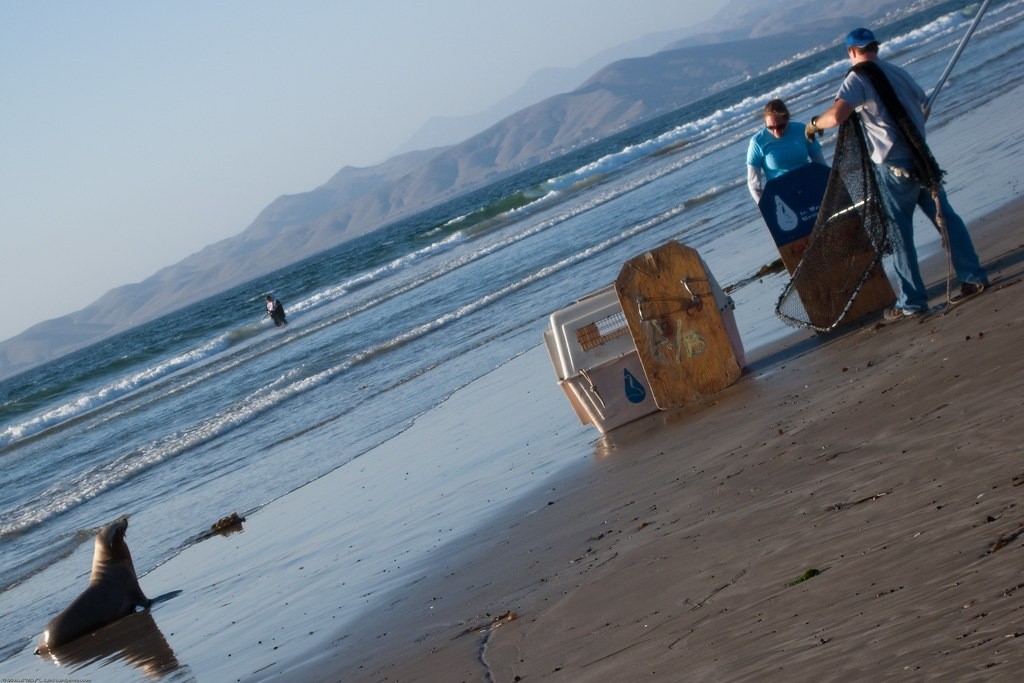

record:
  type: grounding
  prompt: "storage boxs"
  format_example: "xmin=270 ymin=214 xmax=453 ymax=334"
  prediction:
xmin=541 ymin=238 xmax=747 ymax=435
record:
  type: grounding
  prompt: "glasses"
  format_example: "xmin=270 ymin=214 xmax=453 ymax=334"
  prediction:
xmin=764 ymin=123 xmax=789 ymax=131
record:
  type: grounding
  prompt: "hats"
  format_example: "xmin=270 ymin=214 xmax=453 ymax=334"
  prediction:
xmin=845 ymin=28 xmax=883 ymax=50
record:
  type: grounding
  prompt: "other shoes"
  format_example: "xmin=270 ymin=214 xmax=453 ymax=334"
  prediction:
xmin=883 ymin=304 xmax=923 ymax=320
xmin=949 ymin=282 xmax=988 ymax=304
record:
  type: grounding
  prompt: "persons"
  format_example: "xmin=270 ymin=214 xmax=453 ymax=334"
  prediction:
xmin=264 ymin=296 xmax=287 ymax=326
xmin=747 ymin=100 xmax=827 ymax=204
xmin=805 ymin=27 xmax=988 ymax=321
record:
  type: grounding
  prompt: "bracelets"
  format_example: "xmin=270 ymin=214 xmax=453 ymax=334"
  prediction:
xmin=811 ymin=116 xmax=819 ymax=127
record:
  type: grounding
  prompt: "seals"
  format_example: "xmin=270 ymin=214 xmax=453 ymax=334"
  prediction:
xmin=30 ymin=517 xmax=186 ymax=655
